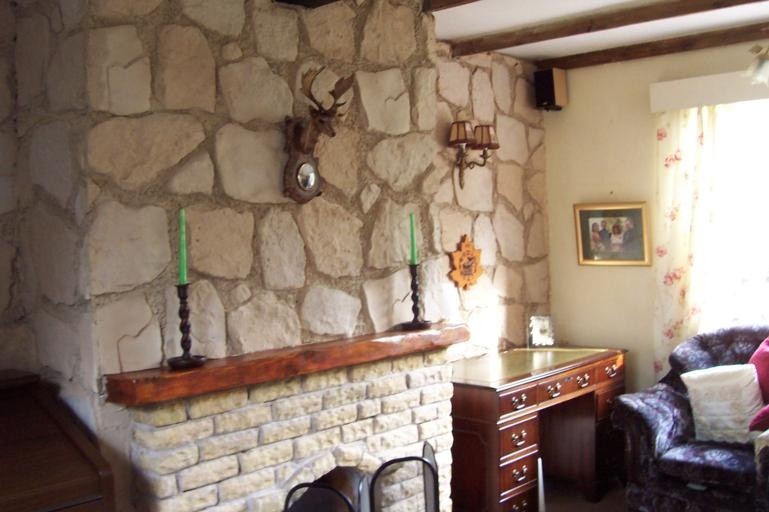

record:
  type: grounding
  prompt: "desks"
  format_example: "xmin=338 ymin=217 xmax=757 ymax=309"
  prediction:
xmin=449 ymin=340 xmax=632 ymax=511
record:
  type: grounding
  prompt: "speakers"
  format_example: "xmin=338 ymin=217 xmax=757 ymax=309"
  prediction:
xmin=534 ymin=67 xmax=566 ymax=108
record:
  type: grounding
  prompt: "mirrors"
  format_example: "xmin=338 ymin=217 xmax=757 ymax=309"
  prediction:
xmin=282 ymin=64 xmax=355 ymax=205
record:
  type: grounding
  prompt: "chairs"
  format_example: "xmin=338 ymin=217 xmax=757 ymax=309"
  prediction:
xmin=603 ymin=322 xmax=769 ymax=512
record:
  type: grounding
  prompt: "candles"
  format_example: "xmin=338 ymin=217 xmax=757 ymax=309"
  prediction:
xmin=177 ymin=207 xmax=189 ymax=284
xmin=409 ymin=210 xmax=416 ymax=265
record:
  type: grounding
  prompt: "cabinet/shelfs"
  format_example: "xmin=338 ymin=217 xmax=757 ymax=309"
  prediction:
xmin=0 ymin=371 xmax=117 ymax=511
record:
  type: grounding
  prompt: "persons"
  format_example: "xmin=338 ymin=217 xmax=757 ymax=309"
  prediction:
xmin=591 ymin=219 xmax=634 ymax=259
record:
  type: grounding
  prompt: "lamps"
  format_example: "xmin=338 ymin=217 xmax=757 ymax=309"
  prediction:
xmin=447 ymin=119 xmax=502 ymax=190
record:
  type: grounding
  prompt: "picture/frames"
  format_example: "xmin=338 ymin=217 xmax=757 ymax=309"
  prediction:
xmin=572 ymin=201 xmax=652 ymax=267
xmin=527 ymin=313 xmax=558 ymax=347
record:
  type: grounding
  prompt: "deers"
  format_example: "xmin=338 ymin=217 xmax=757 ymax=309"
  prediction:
xmin=296 ymin=64 xmax=356 ymax=154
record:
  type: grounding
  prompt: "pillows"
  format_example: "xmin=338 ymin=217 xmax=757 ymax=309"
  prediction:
xmin=746 ymin=334 xmax=769 ymax=406
xmin=676 ymin=360 xmax=766 ymax=446
xmin=748 ymin=400 xmax=769 ymax=434
